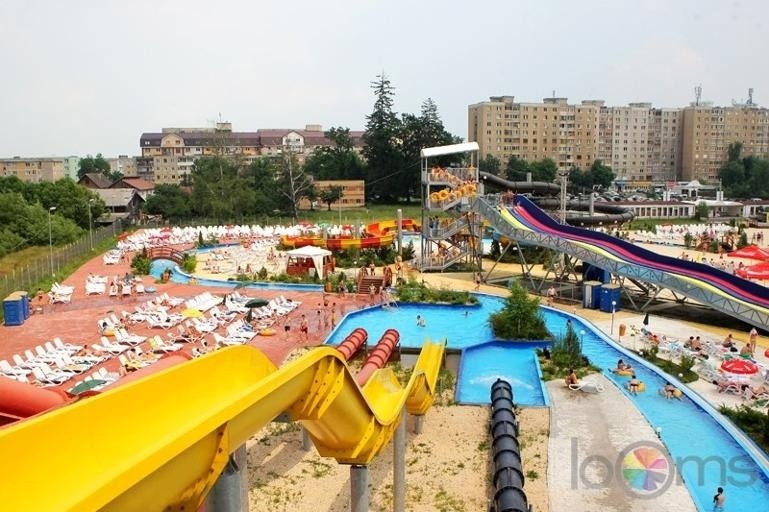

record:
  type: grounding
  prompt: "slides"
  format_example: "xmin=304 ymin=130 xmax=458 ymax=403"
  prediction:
xmin=0 ymin=328 xmax=447 ymax=512
xmin=478 ymin=170 xmax=635 ymax=227
xmin=281 ymin=219 xmax=421 ymax=252
xmin=489 ymin=377 xmax=532 ymax=512
xmin=470 ymin=195 xmax=769 ymax=332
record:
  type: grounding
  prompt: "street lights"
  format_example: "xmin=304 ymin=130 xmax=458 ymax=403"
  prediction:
xmin=88 ymin=198 xmax=94 ymax=251
xmin=49 ymin=206 xmax=57 ymax=277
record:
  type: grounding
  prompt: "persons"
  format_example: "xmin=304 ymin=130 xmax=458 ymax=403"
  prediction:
xmin=682 ymin=328 xmax=758 ymax=359
xmin=497 ymin=189 xmax=521 ymax=214
xmin=48 ymin=291 xmax=56 ymax=305
xmin=652 ymin=334 xmax=662 ymax=344
xmin=567 ymin=368 xmax=577 ymax=389
xmin=616 ymin=360 xmax=642 ymax=395
xmin=546 ymin=285 xmax=557 ymax=308
xmin=37 ymin=288 xmax=43 ymax=302
xmin=600 ymin=223 xmax=768 ymax=286
xmin=27 ymin=227 xmax=405 ymax=387
xmin=462 ymin=311 xmax=471 ymax=316
xmin=664 ymin=381 xmax=683 ymax=402
xmin=475 ymin=272 xmax=481 ymax=292
xmin=29 ymin=300 xmax=34 ymax=315
xmin=714 ymin=380 xmax=769 ymax=396
xmin=430 ymin=165 xmax=485 ymax=266
xmin=416 ymin=315 xmax=425 ymax=327
xmin=713 ymin=487 xmax=724 ymax=511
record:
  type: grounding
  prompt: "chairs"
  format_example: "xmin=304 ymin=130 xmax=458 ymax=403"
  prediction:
xmin=0 ymin=273 xmax=304 ymax=398
xmin=637 ymin=331 xmax=769 ymax=412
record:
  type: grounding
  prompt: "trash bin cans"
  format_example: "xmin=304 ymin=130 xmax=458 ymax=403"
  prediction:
xmin=583 ymin=281 xmax=620 ymax=313
xmin=3 ymin=291 xmax=30 ymax=326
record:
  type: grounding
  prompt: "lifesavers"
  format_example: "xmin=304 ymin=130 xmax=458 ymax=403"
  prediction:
xmin=659 ymin=386 xmax=683 ymax=398
xmin=623 ymin=381 xmax=645 ymax=392
xmin=428 ymin=166 xmax=477 ymax=265
xmin=615 ymin=367 xmax=633 ymax=375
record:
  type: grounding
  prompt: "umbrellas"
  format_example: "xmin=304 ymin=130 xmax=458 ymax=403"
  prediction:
xmin=719 ymin=358 xmax=759 ymax=389
